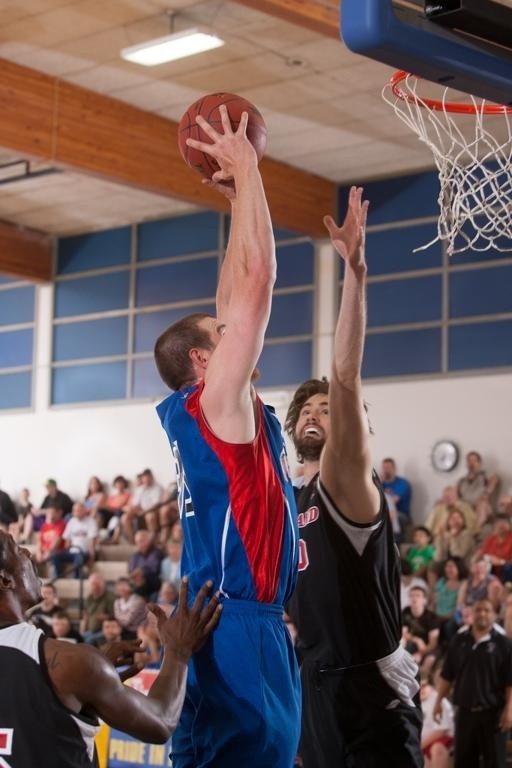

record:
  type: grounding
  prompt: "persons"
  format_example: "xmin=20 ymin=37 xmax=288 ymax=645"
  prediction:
xmin=154 ymin=103 xmax=300 ymax=764
xmin=285 ymin=183 xmax=422 ymax=764
xmin=0 ymin=452 xmax=510 ymax=766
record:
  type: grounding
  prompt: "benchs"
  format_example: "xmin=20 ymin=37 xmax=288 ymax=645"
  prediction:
xmin=38 ymin=542 xmax=137 ymax=600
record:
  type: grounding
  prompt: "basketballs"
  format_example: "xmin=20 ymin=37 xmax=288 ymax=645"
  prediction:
xmin=178 ymin=93 xmax=267 ymax=184
